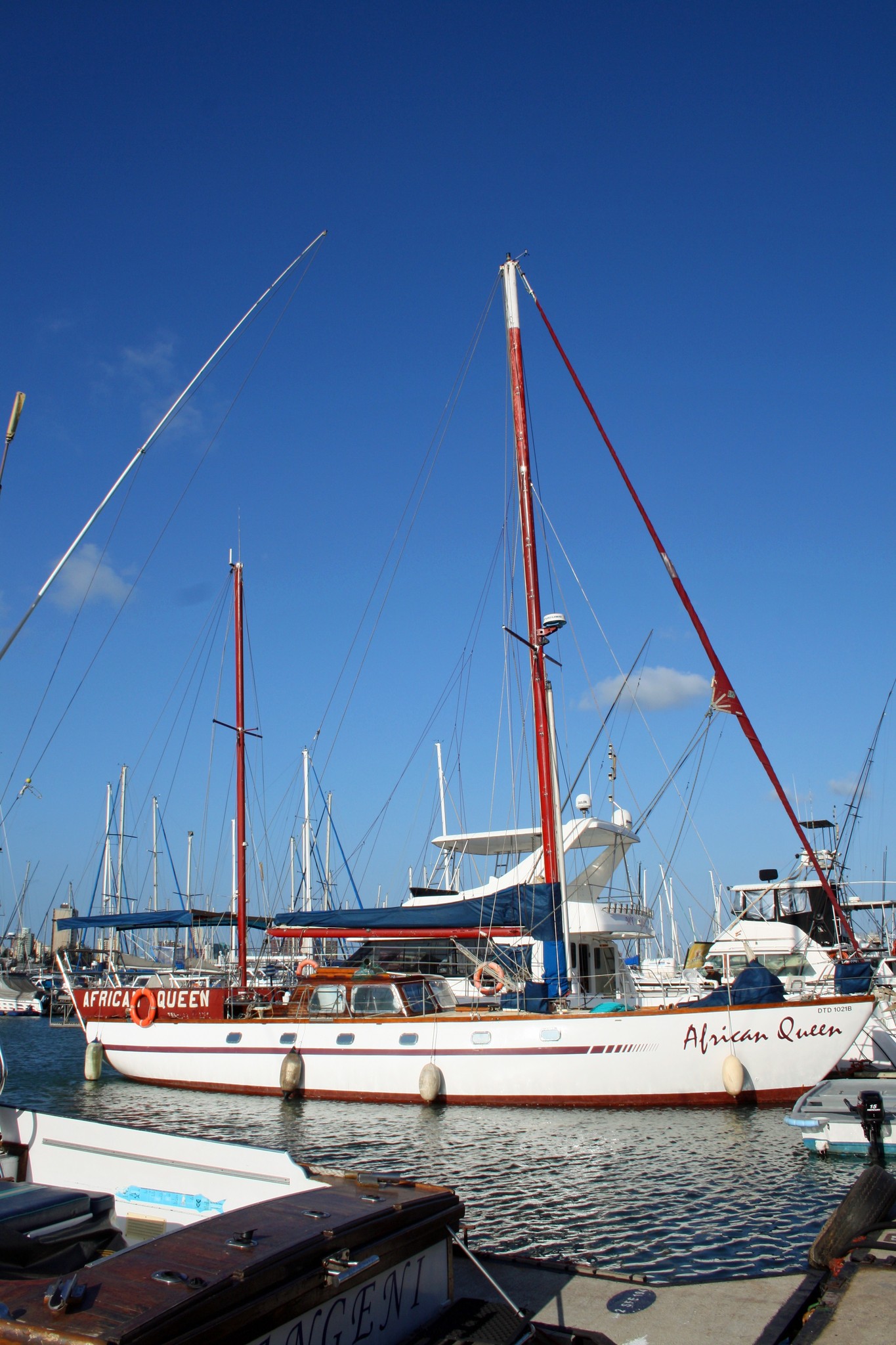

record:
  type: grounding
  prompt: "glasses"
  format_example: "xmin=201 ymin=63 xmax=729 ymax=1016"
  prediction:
xmin=705 ymin=968 xmax=708 ymax=970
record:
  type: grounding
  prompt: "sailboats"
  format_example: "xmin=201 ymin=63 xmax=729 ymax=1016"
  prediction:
xmin=57 ymin=248 xmax=895 ymax=1109
xmin=0 ymin=544 xmax=896 ymax=1078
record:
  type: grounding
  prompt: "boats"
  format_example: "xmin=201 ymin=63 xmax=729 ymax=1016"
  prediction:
xmin=0 ymin=1054 xmax=618 ymax=1345
xmin=782 ymin=1079 xmax=895 ymax=1170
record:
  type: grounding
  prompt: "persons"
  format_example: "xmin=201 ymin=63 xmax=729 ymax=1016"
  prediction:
xmin=702 ymin=962 xmax=722 ymax=990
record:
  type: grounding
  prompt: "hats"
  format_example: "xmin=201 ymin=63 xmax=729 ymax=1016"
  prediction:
xmin=702 ymin=962 xmax=714 ymax=968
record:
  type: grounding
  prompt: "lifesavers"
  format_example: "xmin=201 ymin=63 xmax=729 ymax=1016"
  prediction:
xmin=828 ymin=951 xmax=850 ymax=963
xmin=130 ymin=987 xmax=156 ymax=1027
xmin=892 ymin=940 xmax=896 ymax=956
xmin=473 ymin=961 xmax=504 ymax=996
xmin=296 ymin=958 xmax=319 ymax=977
